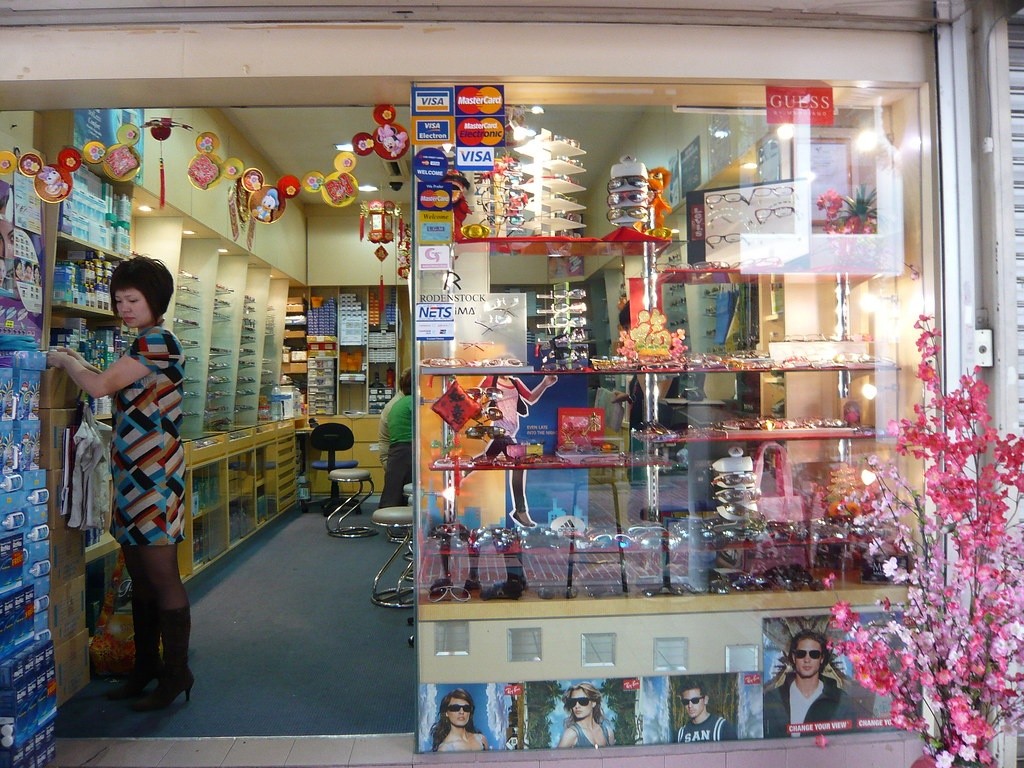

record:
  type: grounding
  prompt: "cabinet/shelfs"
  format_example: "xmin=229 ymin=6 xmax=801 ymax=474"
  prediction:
xmin=34 ymin=107 xmax=145 ymax=352
xmin=306 ymin=415 xmax=385 ymax=496
xmin=177 ymin=419 xmax=302 ymax=583
xmin=131 ymin=217 xmax=288 ymax=446
xmin=281 ymin=285 xmax=411 ymax=416
xmin=407 ymin=77 xmax=931 ymax=755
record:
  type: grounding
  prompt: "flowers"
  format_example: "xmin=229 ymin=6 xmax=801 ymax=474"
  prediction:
xmin=817 ymin=181 xmax=877 ymax=234
xmin=828 ymin=365 xmax=1024 ymax=768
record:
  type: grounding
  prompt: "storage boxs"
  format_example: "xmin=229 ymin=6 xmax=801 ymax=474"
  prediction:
xmin=0 ymin=333 xmax=58 ymax=768
xmin=52 ymin=251 xmax=114 ymax=312
xmin=50 ymin=317 xmax=139 ymax=415
xmin=39 ymin=365 xmax=91 ymax=707
xmin=58 ymin=163 xmax=133 ymax=257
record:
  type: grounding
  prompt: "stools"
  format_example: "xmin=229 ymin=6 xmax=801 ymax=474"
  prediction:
xmin=325 ymin=469 xmax=380 ymax=537
xmin=370 ymin=506 xmax=414 ymax=609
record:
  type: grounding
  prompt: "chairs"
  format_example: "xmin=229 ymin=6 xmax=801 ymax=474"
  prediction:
xmin=302 ymin=422 xmax=362 ymax=517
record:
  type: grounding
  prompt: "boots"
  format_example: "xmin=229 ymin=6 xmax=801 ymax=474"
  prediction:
xmin=106 ymin=584 xmax=165 ymax=701
xmin=133 ymin=601 xmax=195 ymax=712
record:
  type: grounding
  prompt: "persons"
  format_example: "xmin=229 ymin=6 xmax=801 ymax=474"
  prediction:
xmin=557 ymin=683 xmax=614 ymax=747
xmin=610 ymin=300 xmax=689 ymax=526
xmin=678 ymin=680 xmax=738 ymax=744
xmin=46 ymin=253 xmax=195 ymax=711
xmin=430 ymin=688 xmax=490 ymax=753
xmin=763 ymin=628 xmax=873 ymax=739
xmin=379 ymin=368 xmax=414 ymax=509
xmin=461 ymin=375 xmax=558 ymax=527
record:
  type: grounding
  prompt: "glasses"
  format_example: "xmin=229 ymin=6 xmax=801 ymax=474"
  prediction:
xmin=793 ymin=649 xmax=822 ymax=659
xmin=447 ymin=704 xmax=472 ymax=712
xmin=426 ymin=119 xmax=942 ymax=607
xmin=175 ymin=270 xmax=261 ymax=418
xmin=569 ymin=696 xmax=594 ymax=707
xmin=681 ymin=697 xmax=704 ymax=706
